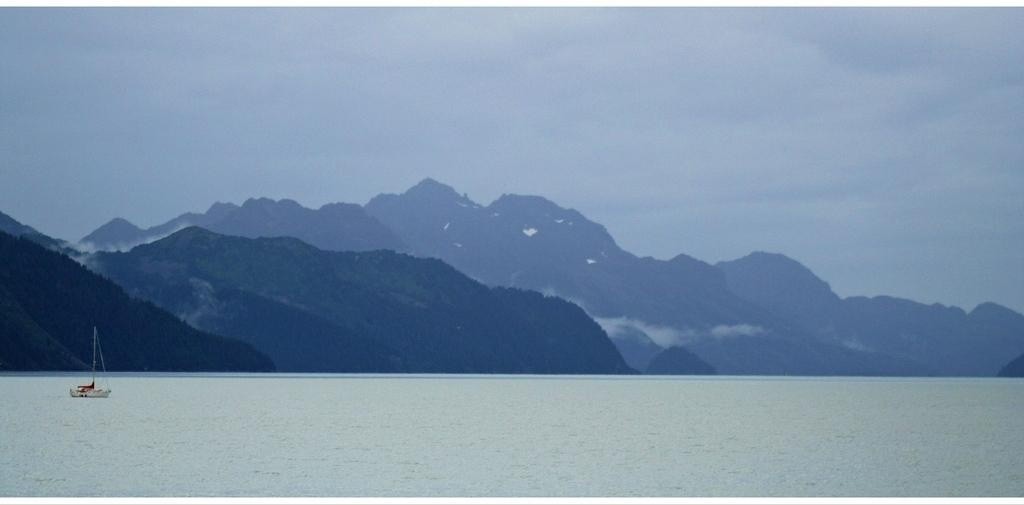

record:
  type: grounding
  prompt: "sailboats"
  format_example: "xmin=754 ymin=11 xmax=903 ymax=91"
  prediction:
xmin=64 ymin=323 xmax=114 ymax=400
